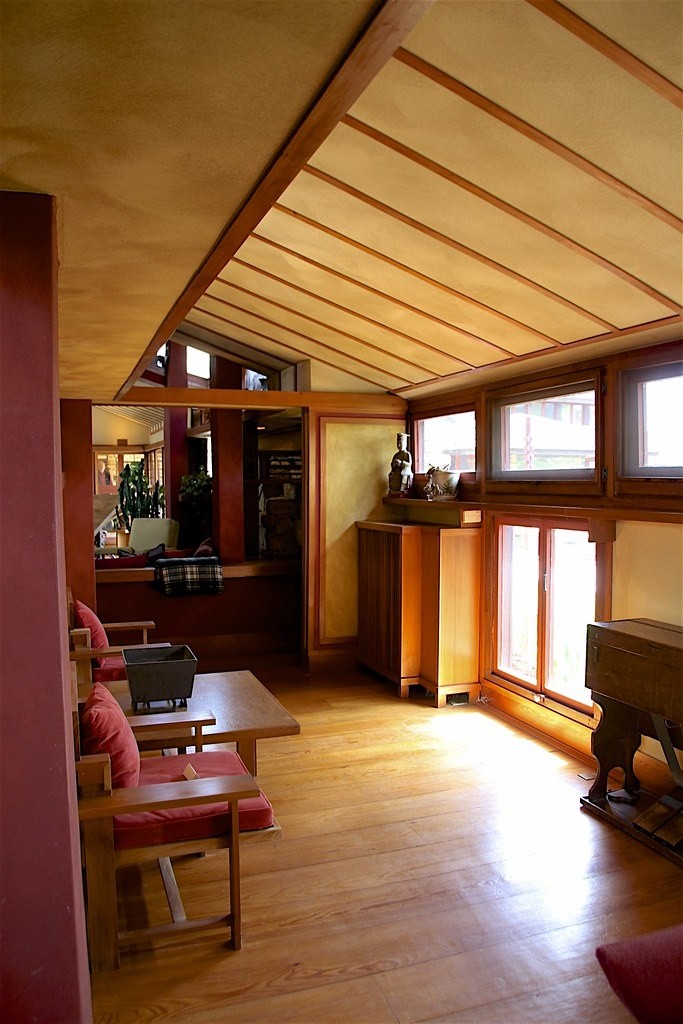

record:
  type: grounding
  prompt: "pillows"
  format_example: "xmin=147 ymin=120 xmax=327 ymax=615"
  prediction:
xmin=95 ymin=555 xmax=149 ymax=571
xmin=95 ymin=547 xmax=135 ymax=555
xmin=162 ymin=548 xmax=195 ymax=558
xmin=192 ymin=537 xmax=215 ymax=557
xmin=71 ymin=598 xmax=112 ymax=669
xmin=77 ymin=682 xmax=141 ymax=793
xmin=117 ymin=543 xmax=165 ymax=558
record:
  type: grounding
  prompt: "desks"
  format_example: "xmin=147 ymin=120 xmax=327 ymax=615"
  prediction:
xmin=79 ymin=670 xmax=302 ymax=779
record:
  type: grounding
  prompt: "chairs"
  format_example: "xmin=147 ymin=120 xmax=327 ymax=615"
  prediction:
xmin=62 ymin=581 xmax=284 ymax=976
xmin=598 ymin=921 xmax=683 ymax=1024
xmin=261 ymin=497 xmax=300 ymax=561
xmin=127 ymin=518 xmax=180 ymax=555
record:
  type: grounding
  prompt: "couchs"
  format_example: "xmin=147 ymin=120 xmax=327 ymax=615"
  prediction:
xmin=95 ymin=561 xmax=299 ymax=659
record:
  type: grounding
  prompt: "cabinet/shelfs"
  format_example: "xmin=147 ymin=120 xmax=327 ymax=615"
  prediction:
xmin=356 ymin=519 xmax=483 ymax=708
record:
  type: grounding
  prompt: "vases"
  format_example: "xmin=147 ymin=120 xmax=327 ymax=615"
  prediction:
xmin=437 ymin=471 xmax=460 ymax=495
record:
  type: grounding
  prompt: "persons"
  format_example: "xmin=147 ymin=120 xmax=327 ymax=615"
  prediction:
xmin=389 ymin=432 xmax=412 ymax=491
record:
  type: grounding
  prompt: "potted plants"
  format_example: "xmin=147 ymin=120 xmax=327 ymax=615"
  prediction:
xmin=112 ymin=459 xmax=160 ymax=549
xmin=178 ymin=466 xmax=213 ymax=504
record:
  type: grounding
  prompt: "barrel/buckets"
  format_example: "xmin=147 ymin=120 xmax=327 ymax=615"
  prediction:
xmin=432 ymin=471 xmax=460 ymax=496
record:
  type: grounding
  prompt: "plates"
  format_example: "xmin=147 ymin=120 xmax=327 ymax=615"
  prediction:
xmin=433 ymin=496 xmax=457 ymax=501
